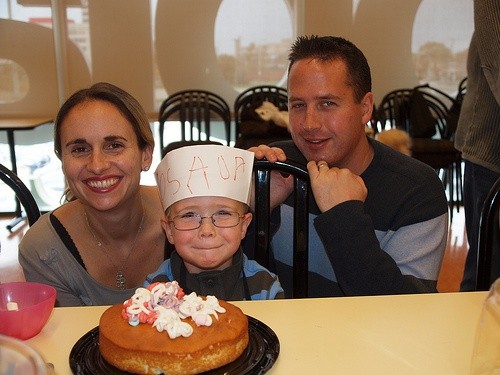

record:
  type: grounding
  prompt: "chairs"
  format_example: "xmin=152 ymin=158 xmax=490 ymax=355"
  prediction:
xmin=0 ymin=76 xmax=500 ymax=299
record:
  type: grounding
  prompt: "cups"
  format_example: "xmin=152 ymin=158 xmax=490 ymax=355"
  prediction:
xmin=471 ymin=277 xmax=500 ymax=375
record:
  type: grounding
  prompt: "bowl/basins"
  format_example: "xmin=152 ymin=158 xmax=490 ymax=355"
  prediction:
xmin=0 ymin=281 xmax=56 ymax=341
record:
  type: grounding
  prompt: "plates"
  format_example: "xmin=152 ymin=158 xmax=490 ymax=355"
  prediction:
xmin=68 ymin=314 xmax=280 ymax=375
xmin=0 ymin=334 xmax=49 ymax=375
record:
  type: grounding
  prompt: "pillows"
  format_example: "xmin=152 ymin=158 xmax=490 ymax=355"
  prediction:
xmin=239 ymin=103 xmax=289 ymax=137
xmin=400 ymin=91 xmax=436 ymax=139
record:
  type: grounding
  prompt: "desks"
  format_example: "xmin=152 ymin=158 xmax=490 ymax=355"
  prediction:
xmin=0 ymin=117 xmax=53 ymax=234
xmin=24 ymin=290 xmax=488 ymax=375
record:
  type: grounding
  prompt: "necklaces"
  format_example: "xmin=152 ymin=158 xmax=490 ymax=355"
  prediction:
xmin=84 ymin=208 xmax=146 ymax=291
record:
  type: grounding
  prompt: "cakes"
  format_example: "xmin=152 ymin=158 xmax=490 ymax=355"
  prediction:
xmin=98 ymin=280 xmax=248 ymax=375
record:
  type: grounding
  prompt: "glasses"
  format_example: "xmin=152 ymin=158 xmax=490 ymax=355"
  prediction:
xmin=164 ymin=211 xmax=245 ymax=231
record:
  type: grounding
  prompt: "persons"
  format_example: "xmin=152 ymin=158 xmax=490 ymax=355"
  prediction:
xmin=18 ymin=82 xmax=170 ymax=308
xmin=245 ymin=35 xmax=448 ymax=304
xmin=142 ymin=145 xmax=286 ymax=302
xmin=454 ymin=0 xmax=500 ymax=292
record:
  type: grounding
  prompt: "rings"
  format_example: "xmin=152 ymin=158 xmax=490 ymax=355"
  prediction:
xmin=318 ymin=163 xmax=329 ymax=167
